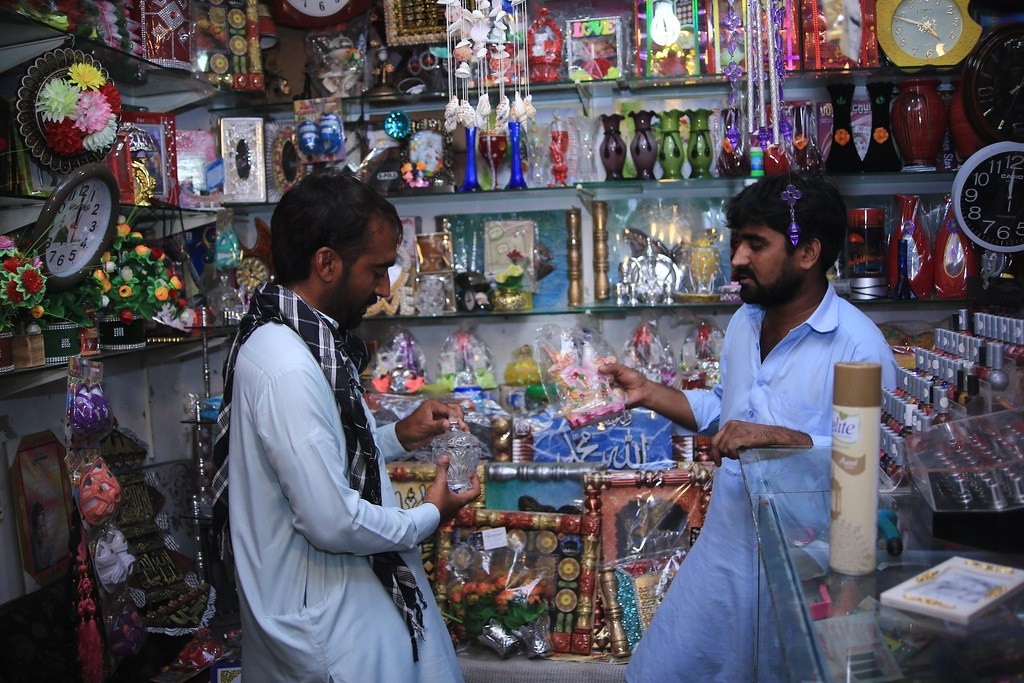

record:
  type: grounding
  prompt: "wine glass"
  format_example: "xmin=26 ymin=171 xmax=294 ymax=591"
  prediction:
xmin=326 ymin=47 xmax=356 ymax=97
xmin=478 ymin=131 xmax=507 ymax=190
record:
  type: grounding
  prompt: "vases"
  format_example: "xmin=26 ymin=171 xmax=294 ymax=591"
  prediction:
xmin=892 ymin=78 xmax=945 ymax=173
xmin=890 ymin=193 xmax=978 ymax=299
xmin=496 ymin=288 xmax=525 ymax=310
xmin=946 ymin=70 xmax=981 ymax=162
xmin=600 ymin=80 xmax=901 ymax=183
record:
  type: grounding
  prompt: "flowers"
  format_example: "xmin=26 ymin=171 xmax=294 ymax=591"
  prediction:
xmin=493 ymin=250 xmax=522 ymax=288
xmin=34 ymin=60 xmax=120 ymax=156
xmin=0 ymin=203 xmax=187 ymax=335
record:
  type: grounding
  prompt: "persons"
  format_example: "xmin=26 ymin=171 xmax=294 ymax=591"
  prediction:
xmin=210 ymin=170 xmax=481 ymax=683
xmin=598 ymin=168 xmax=901 ymax=683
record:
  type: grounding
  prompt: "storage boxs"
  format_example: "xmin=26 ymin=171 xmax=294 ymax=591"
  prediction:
xmin=400 ymin=217 xmax=422 ymax=248
xmin=412 ymin=229 xmax=458 ymax=319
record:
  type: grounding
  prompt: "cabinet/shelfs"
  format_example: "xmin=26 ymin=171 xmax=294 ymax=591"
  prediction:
xmin=0 ymin=0 xmax=252 ymax=398
xmin=209 ymin=65 xmax=983 ymax=319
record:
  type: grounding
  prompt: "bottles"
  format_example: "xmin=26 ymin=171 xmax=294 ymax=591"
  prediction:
xmin=749 ymin=136 xmax=765 ymax=178
xmin=527 ymin=109 xmax=714 ymax=188
xmin=826 ymin=79 xmax=986 ymax=174
xmin=828 ymin=361 xmax=882 ymax=576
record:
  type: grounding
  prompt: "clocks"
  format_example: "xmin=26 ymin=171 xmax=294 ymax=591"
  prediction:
xmin=34 ymin=165 xmax=122 ymax=292
xmin=874 ymin=0 xmax=1024 ymax=253
xmin=456 ymin=272 xmax=494 ymax=311
xmin=269 ymin=0 xmax=370 ymax=28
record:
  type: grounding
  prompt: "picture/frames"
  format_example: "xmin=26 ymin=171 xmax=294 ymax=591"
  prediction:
xmin=119 ymin=111 xmax=178 ymax=208
xmin=385 ymin=461 xmax=716 ymax=657
xmin=381 ymin=1 xmax=479 ymax=47
xmin=881 ymin=556 xmax=1024 ymax=625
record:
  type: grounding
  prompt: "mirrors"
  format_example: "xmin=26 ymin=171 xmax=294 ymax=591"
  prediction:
xmin=362 ymin=243 xmax=414 ymax=317
xmin=273 ymin=125 xmax=306 ymax=194
